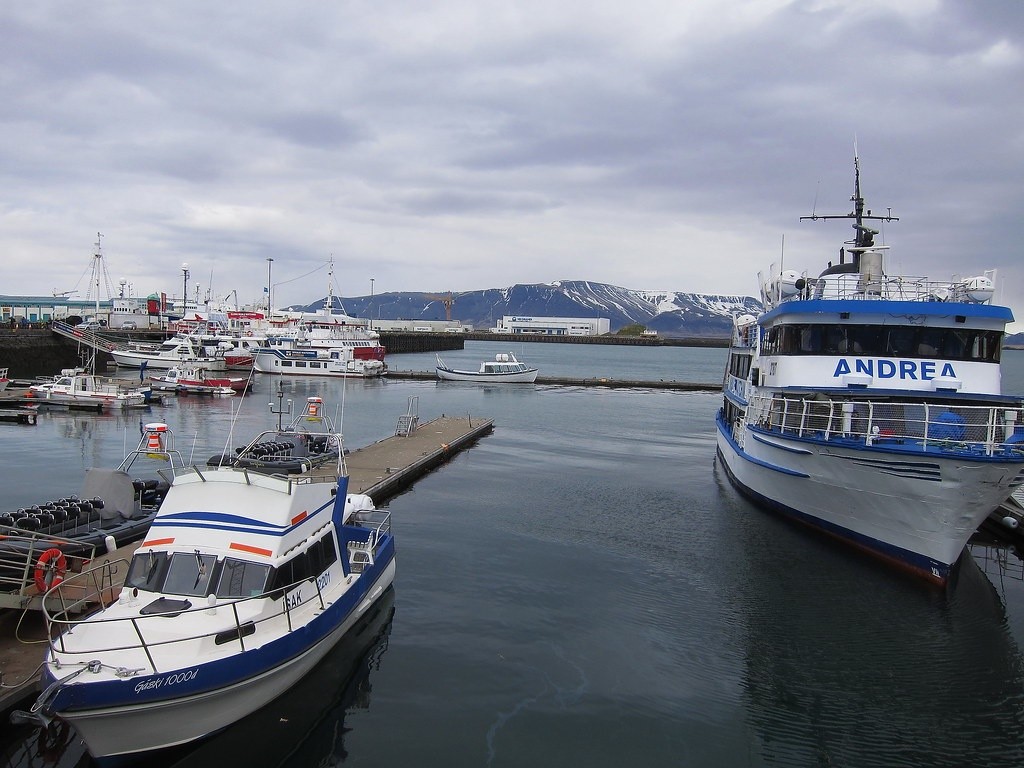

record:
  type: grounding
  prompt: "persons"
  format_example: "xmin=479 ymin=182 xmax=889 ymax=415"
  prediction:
xmin=22 ymin=317 xmax=27 ymax=328
xmin=11 ymin=317 xmax=15 ymax=328
xmin=258 ymin=569 xmax=283 ymax=596
xmin=48 ymin=318 xmax=52 ymax=329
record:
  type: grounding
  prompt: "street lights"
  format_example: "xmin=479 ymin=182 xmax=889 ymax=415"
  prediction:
xmin=370 ymin=279 xmax=375 ymax=331
xmin=181 ymin=263 xmax=191 ymax=318
xmin=120 ymin=277 xmax=127 ymax=299
xmin=266 ymin=258 xmax=274 ymax=318
xmin=196 ymin=283 xmax=200 ymax=304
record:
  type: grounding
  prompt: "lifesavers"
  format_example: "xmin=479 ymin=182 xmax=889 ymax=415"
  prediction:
xmin=742 ymin=326 xmax=749 ymax=346
xmin=34 ymin=548 xmax=66 ymax=593
xmin=247 ymin=330 xmax=252 ymax=337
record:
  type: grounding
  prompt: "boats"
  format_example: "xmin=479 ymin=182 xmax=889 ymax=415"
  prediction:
xmin=434 ymin=345 xmax=538 ymax=383
xmin=29 ymin=353 xmax=397 ymax=768
xmin=204 ymin=375 xmax=248 ymax=391
xmin=28 ymin=367 xmax=145 ymax=409
xmin=712 ymin=145 xmax=1024 ymax=586
xmin=111 ymin=254 xmax=389 ymax=378
xmin=203 ymin=358 xmax=349 ymax=473
xmin=0 ymin=421 xmax=200 ymax=595
xmin=146 ymin=365 xmax=237 ymax=394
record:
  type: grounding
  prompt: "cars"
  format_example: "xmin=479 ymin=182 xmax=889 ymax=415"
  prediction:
xmin=120 ymin=320 xmax=138 ymax=330
xmin=76 ymin=322 xmax=102 ymax=330
